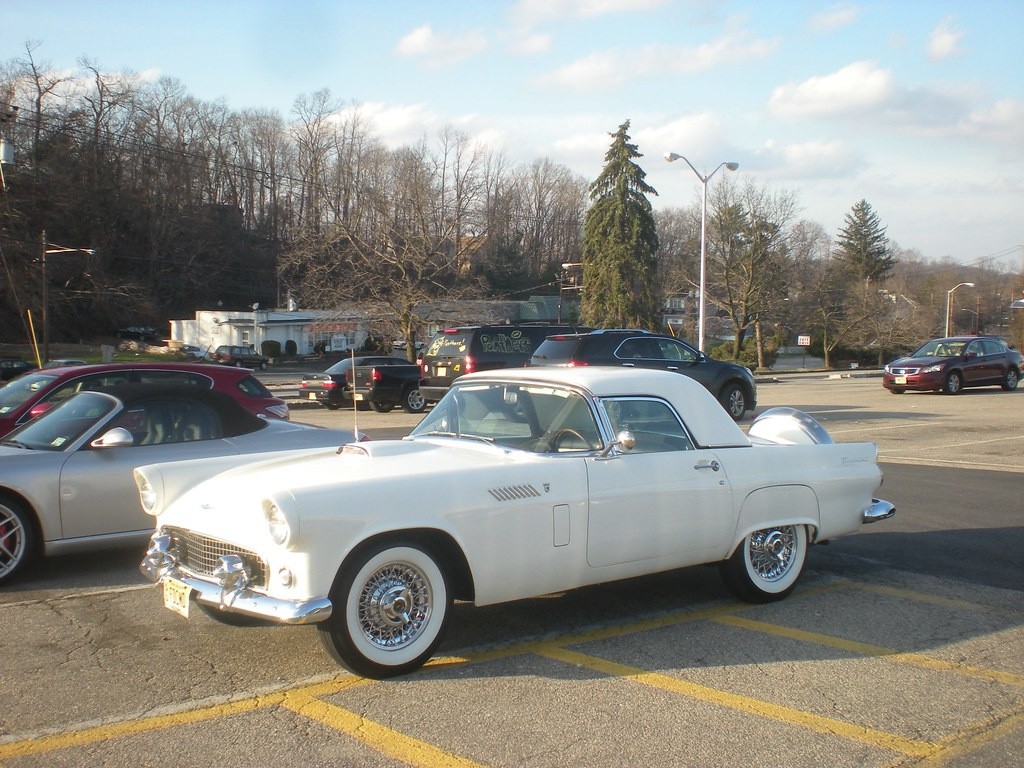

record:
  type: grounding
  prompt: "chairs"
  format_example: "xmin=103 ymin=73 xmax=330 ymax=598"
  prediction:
xmin=173 ymin=402 xmax=221 ymax=441
xmin=140 ymin=398 xmax=175 ymax=445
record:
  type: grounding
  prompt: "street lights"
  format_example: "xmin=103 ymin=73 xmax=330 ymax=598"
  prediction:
xmin=945 ymin=283 xmax=975 ymax=338
xmin=962 ymin=308 xmax=978 ymax=336
xmin=558 ymin=285 xmax=588 ymax=326
xmin=42 ymin=249 xmax=98 ymax=362
xmin=998 ymin=293 xmax=1013 ymax=324
xmin=664 ymin=152 xmax=738 ymax=353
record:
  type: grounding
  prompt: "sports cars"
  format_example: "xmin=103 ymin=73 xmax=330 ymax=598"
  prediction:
xmin=0 ymin=388 xmax=373 ymax=585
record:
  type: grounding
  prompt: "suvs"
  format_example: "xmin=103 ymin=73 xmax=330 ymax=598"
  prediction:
xmin=0 ymin=364 xmax=290 ymax=436
xmin=418 ymin=323 xmax=599 ymax=422
xmin=523 ymin=328 xmax=757 ymax=428
xmin=214 ymin=345 xmax=272 ymax=371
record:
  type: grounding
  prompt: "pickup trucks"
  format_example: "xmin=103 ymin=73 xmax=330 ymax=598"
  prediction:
xmin=343 ymin=366 xmax=437 ymax=413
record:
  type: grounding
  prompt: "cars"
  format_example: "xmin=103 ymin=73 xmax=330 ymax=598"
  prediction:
xmin=40 ymin=360 xmax=87 ymax=369
xmin=882 ymin=335 xmax=1024 ymax=394
xmin=392 ymin=338 xmax=425 ymax=350
xmin=133 ymin=365 xmax=899 ymax=678
xmin=180 ymin=344 xmax=210 ymax=360
xmin=0 ymin=359 xmax=34 ymax=381
xmin=299 ymin=355 xmax=420 ymax=410
xmin=116 ymin=325 xmax=159 ymax=342
